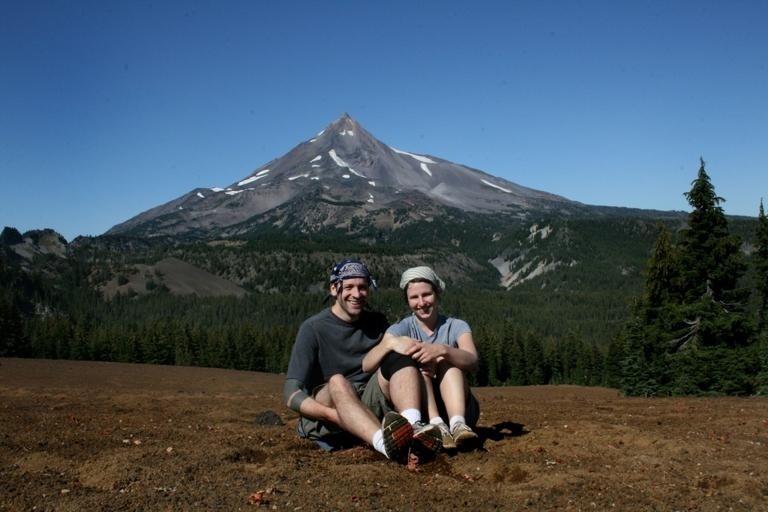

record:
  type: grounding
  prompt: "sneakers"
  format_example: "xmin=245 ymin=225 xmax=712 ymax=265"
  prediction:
xmin=452 ymin=420 xmax=479 ymax=450
xmin=412 ymin=420 xmax=443 ymax=464
xmin=431 ymin=423 xmax=457 ymax=452
xmin=379 ymin=411 xmax=414 ymax=466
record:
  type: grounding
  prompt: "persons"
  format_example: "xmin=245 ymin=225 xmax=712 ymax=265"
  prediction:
xmin=282 ymin=259 xmax=443 ymax=470
xmin=361 ymin=266 xmax=480 ymax=452
